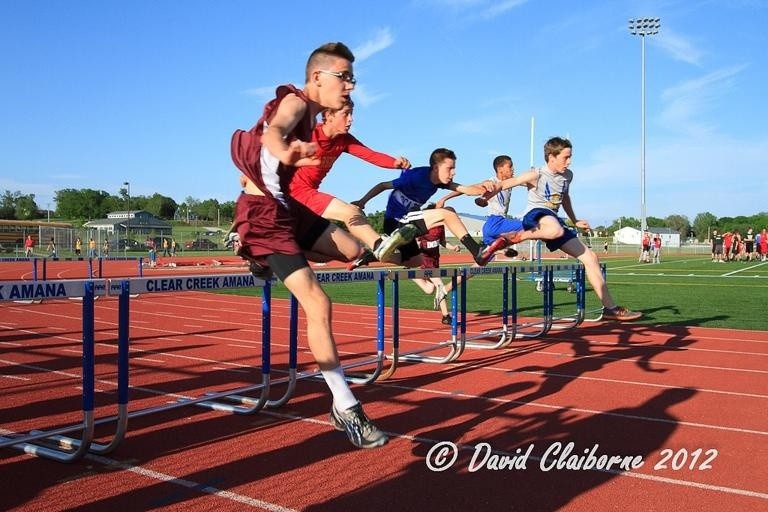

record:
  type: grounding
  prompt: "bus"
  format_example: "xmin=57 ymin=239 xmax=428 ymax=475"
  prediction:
xmin=0 ymin=219 xmax=76 ymax=248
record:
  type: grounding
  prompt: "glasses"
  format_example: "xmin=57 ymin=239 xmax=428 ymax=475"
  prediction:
xmin=317 ymin=68 xmax=357 ymax=84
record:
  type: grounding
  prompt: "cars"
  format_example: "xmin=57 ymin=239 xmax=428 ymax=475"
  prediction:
xmin=108 ymin=239 xmax=147 ymax=251
xmin=0 ymin=243 xmax=13 ymax=253
xmin=144 ymin=237 xmax=179 ymax=249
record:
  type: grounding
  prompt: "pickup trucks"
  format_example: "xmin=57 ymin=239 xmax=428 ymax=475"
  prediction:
xmin=184 ymin=238 xmax=218 ymax=250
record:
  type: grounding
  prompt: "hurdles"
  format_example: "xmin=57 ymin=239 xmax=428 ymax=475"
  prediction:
xmin=0 ymin=257 xmax=607 ymax=463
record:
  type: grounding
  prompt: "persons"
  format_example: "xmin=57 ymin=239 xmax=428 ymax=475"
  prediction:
xmin=636 ymin=231 xmax=651 ymax=263
xmin=602 ymin=240 xmax=608 ymax=254
xmin=228 ymin=42 xmax=389 ymax=448
xmin=710 ymin=226 xmax=768 ymax=264
xmin=25 ymin=235 xmax=179 ymax=260
xmin=239 ymin=95 xmax=418 ymax=278
xmin=409 ymin=202 xmax=462 ymax=326
xmin=432 ymin=155 xmax=533 ymax=310
xmin=348 ymin=148 xmax=507 ymax=268
xmin=474 ymin=135 xmax=644 ymax=321
xmin=652 ymin=233 xmax=662 ymax=264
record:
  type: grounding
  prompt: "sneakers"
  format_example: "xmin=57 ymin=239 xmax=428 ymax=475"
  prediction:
xmin=441 ymin=314 xmax=461 ymax=327
xmin=222 ymin=220 xmax=242 ymax=247
xmin=348 ymin=248 xmax=379 ymax=271
xmin=601 ymin=305 xmax=643 ymax=320
xmin=433 ymin=284 xmax=447 ymax=310
xmin=488 ymin=231 xmax=517 ymax=263
xmin=250 ymin=260 xmax=274 ymax=280
xmin=330 ymin=400 xmax=390 ymax=449
xmin=560 ymin=218 xmax=574 ymax=227
xmin=475 ymin=237 xmax=508 ymax=267
xmin=373 ymin=224 xmax=418 ymax=264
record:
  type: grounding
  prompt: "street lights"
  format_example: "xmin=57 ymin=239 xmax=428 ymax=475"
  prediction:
xmin=628 ymin=16 xmax=661 ymax=245
xmin=178 ymin=207 xmax=191 ymax=223
xmin=124 ymin=182 xmax=131 ymax=242
